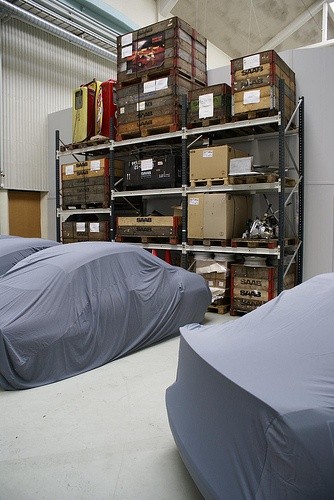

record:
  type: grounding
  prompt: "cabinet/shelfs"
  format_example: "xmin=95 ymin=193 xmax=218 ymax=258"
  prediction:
xmin=55 ymin=16 xmax=304 ymax=316
